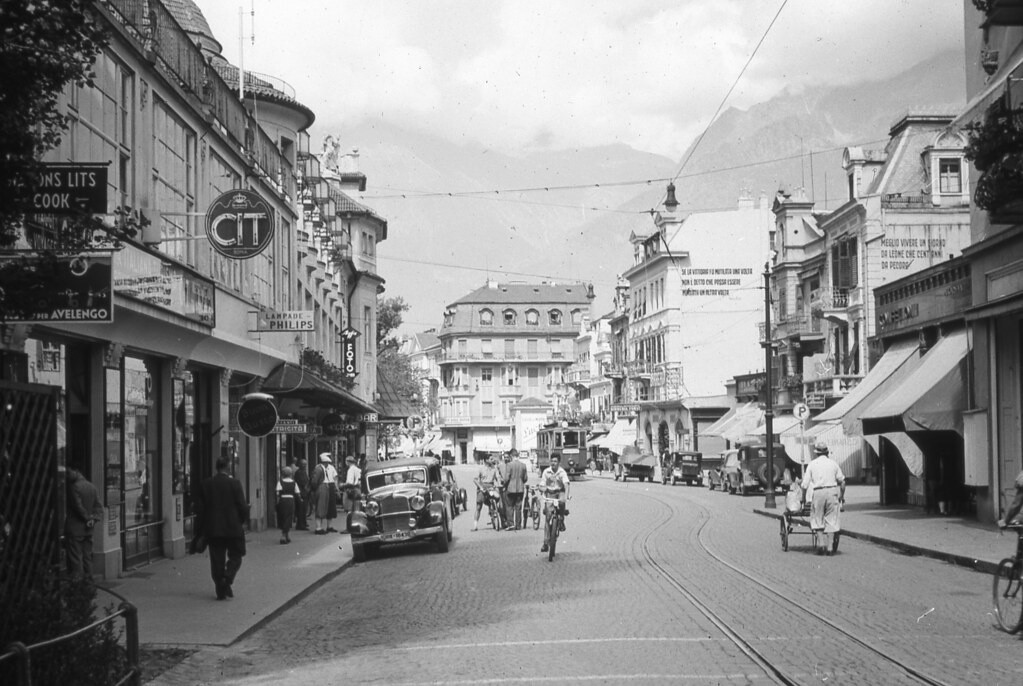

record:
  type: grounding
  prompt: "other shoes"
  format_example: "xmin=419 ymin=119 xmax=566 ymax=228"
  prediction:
xmin=559 ymin=521 xmax=566 ymax=531
xmin=216 ymin=577 xmax=234 ymax=601
xmin=471 ymin=527 xmax=477 ymax=531
xmin=541 ymin=544 xmax=548 ymax=552
xmin=280 ymin=519 xmax=351 ymax=543
xmin=826 ymin=550 xmax=834 ymax=556
xmin=815 ymin=546 xmax=826 ymax=556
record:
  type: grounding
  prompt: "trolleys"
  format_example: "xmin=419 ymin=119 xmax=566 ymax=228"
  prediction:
xmin=776 ymin=495 xmax=847 ymax=556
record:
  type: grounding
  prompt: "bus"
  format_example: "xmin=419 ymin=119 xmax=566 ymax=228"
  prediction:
xmin=535 ymin=420 xmax=590 ymax=480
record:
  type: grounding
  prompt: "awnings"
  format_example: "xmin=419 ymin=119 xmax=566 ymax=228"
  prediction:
xmin=857 ymin=326 xmax=975 ymax=436
xmin=997 ymin=471 xmax=1023 ymax=583
xmin=598 ymin=418 xmax=636 ymax=456
xmin=694 ymin=400 xmax=801 ymax=444
xmin=812 ymin=336 xmax=921 ymax=435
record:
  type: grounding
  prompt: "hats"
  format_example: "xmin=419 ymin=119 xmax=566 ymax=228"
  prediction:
xmin=188 ymin=536 xmax=207 ymax=555
xmin=321 ymin=455 xmax=332 ymax=462
xmin=814 ymin=442 xmax=829 ymax=453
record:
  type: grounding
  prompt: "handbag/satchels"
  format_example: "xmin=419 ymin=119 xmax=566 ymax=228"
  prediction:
xmin=347 ymin=485 xmax=362 ymax=500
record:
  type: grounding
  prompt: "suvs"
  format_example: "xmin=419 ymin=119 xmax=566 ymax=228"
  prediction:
xmin=338 ymin=455 xmax=456 ymax=563
xmin=439 ymin=468 xmax=469 ymax=517
xmin=707 ymin=443 xmax=787 ymax=497
xmin=612 ymin=446 xmax=657 ymax=483
xmin=662 ymin=450 xmax=706 ymax=487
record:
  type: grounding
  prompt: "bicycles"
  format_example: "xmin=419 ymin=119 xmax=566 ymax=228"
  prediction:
xmin=522 ymin=484 xmax=544 ymax=530
xmin=990 ymin=523 xmax=1023 ymax=634
xmin=537 ymin=487 xmax=569 ymax=560
xmin=480 ymin=485 xmax=506 ymax=530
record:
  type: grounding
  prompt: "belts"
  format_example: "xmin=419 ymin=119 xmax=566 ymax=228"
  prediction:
xmin=814 ymin=486 xmax=836 ymax=490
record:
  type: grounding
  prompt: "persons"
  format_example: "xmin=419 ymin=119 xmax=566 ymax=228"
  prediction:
xmin=359 ymin=453 xmax=367 ymax=473
xmin=801 ymin=441 xmax=846 ymax=556
xmin=276 ymin=466 xmax=304 ymax=544
xmin=385 ymin=452 xmax=393 ymax=460
xmin=595 ymin=450 xmax=604 ymax=475
xmin=338 ymin=455 xmax=362 ymax=534
xmin=196 ymin=454 xmax=250 ymax=601
xmin=420 ymin=449 xmax=442 ymax=483
xmin=294 ymin=458 xmax=309 ymax=530
xmin=470 ymin=449 xmax=528 ymax=531
xmin=378 ymin=453 xmax=384 ymax=462
xmin=661 ymin=447 xmax=671 ymax=467
xmin=402 ymin=470 xmax=413 ymax=482
xmin=391 ymin=473 xmax=402 ymax=484
xmin=66 ymin=466 xmax=104 ymax=612
xmin=289 ymin=456 xmax=300 ymax=479
xmin=322 ymin=134 xmax=341 ymax=171
xmin=539 ymin=452 xmax=570 ymax=552
xmin=310 ymin=452 xmax=340 ymax=535
